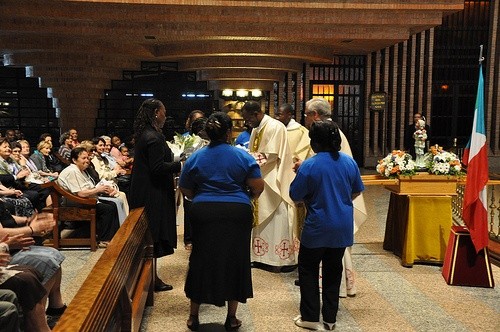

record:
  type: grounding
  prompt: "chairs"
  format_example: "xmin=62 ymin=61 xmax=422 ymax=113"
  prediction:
xmin=41 ymin=180 xmax=98 ymax=253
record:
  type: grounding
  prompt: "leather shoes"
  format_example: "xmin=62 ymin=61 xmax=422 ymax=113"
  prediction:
xmin=154 ymin=281 xmax=173 ymax=290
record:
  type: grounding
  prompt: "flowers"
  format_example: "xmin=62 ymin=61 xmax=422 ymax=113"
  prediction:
xmin=423 ymin=144 xmax=465 ymax=176
xmin=165 ymin=131 xmax=202 ymax=161
xmin=376 ymin=150 xmax=420 ymax=178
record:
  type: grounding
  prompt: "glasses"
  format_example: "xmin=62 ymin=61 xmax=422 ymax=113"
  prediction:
xmin=303 ymin=112 xmax=312 ymax=117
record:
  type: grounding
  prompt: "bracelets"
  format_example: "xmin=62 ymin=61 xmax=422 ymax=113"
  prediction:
xmin=29 ymin=226 xmax=34 ymax=236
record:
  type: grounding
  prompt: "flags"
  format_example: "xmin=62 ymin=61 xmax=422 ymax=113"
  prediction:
xmin=460 ymin=66 xmax=489 ymax=253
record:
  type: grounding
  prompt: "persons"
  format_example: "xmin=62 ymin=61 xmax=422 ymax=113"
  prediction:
xmin=288 ymin=119 xmax=365 ymax=331
xmin=236 ymin=120 xmax=254 ymax=149
xmin=292 ymin=98 xmax=368 ymax=299
xmin=276 ymin=102 xmax=317 ymax=163
xmin=403 ymin=113 xmax=432 ymax=161
xmin=239 ymin=100 xmax=307 ymax=272
xmin=179 ymin=110 xmax=208 ymax=251
xmin=126 ymin=99 xmax=186 ymax=294
xmin=55 ymin=133 xmax=134 ymax=241
xmin=0 ymin=127 xmax=81 ymax=332
xmin=180 ymin=111 xmax=265 ymax=330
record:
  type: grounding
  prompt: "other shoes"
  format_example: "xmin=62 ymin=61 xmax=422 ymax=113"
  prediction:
xmin=294 ymin=316 xmax=317 ymax=330
xmin=46 ymin=305 xmax=67 ymax=318
xmin=188 ymin=314 xmax=199 ymax=329
xmin=323 ymin=322 xmax=336 ymax=330
xmin=295 ymin=280 xmax=300 ymax=285
xmin=97 ymin=241 xmax=109 ymax=247
xmin=224 ymin=316 xmax=241 ymax=329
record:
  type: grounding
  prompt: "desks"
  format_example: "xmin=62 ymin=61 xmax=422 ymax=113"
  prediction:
xmin=382 ymin=192 xmax=452 ymax=268
